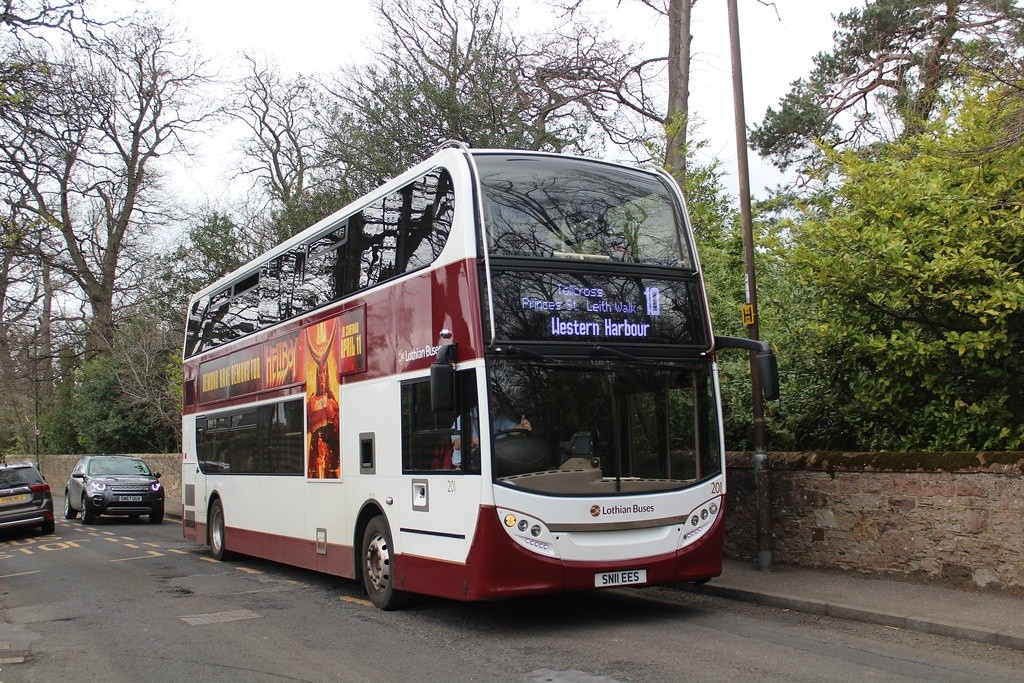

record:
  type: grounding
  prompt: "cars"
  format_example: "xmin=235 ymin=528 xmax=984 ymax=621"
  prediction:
xmin=64 ymin=455 xmax=165 ymax=524
xmin=0 ymin=461 xmax=56 ymax=535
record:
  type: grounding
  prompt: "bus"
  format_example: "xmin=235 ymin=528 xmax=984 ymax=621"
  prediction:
xmin=181 ymin=141 xmax=778 ymax=611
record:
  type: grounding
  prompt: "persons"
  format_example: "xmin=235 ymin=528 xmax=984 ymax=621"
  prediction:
xmin=449 ymin=393 xmax=533 ymax=470
